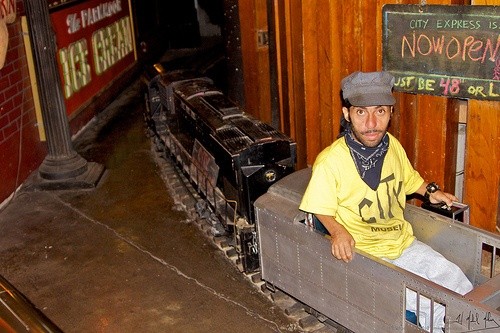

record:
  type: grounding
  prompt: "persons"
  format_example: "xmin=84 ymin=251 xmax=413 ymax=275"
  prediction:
xmin=298 ymin=73 xmax=475 ymax=333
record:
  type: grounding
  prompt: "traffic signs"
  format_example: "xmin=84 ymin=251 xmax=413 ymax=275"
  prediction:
xmin=382 ymin=4 xmax=500 ymax=101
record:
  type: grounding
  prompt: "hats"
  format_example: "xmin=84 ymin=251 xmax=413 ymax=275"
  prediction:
xmin=340 ymin=71 xmax=397 ymax=106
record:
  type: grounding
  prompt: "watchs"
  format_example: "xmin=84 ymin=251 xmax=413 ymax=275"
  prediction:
xmin=425 ymin=181 xmax=440 ymax=200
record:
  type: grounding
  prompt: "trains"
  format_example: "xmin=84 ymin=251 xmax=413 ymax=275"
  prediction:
xmin=141 ymin=57 xmax=499 ymax=333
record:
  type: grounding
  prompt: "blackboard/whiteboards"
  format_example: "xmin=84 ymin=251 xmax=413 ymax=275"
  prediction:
xmin=379 ymin=3 xmax=500 ymax=100
